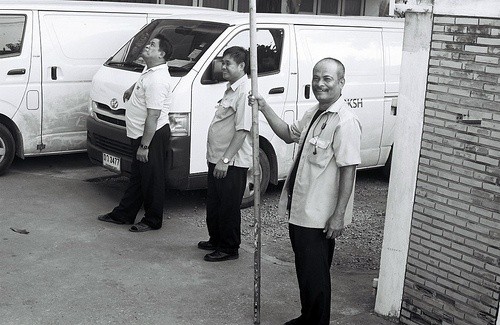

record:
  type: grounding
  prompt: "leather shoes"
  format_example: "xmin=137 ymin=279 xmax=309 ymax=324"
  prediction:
xmin=204 ymin=250 xmax=239 ymax=261
xmin=198 ymin=240 xmax=223 ymax=250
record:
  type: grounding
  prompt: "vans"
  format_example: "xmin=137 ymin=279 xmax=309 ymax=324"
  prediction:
xmin=84 ymin=12 xmax=406 ymax=210
xmin=0 ymin=0 xmax=237 ymax=174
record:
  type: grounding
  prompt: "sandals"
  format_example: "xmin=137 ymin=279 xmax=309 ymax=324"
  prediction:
xmin=98 ymin=212 xmax=125 ymax=224
xmin=129 ymin=222 xmax=154 ymax=232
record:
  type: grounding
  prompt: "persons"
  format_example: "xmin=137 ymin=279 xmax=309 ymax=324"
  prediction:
xmin=198 ymin=46 xmax=252 ymax=262
xmin=248 ymin=57 xmax=362 ymax=325
xmin=98 ymin=33 xmax=172 ymax=232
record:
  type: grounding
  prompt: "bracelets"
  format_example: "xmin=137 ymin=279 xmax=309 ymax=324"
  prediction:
xmin=140 ymin=144 xmax=149 ymax=149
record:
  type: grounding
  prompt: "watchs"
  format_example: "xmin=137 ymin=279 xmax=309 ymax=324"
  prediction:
xmin=221 ymin=157 xmax=232 ymax=164
